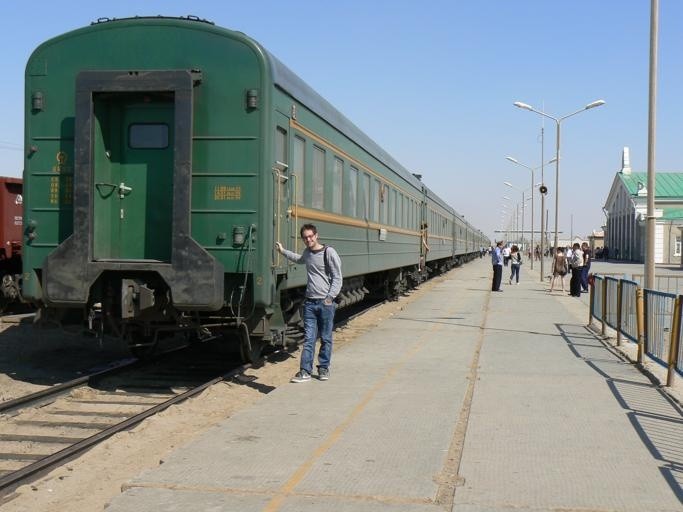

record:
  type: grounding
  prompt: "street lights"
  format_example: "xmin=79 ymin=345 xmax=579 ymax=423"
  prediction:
xmin=497 ymin=155 xmax=558 ymax=270
xmin=515 ymin=100 xmax=605 ymax=276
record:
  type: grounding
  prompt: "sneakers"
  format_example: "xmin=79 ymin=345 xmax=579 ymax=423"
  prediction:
xmin=581 ymin=290 xmax=587 ymax=293
xmin=318 ymin=367 xmax=328 ymax=380
xmin=291 ymin=369 xmax=311 ymax=382
xmin=497 ymin=289 xmax=503 ymax=291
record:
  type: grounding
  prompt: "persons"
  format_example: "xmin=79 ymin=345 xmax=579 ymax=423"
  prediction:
xmin=275 ymin=223 xmax=343 ymax=383
xmin=509 ymin=246 xmax=521 ymax=285
xmin=550 ymin=247 xmax=567 ymax=290
xmin=580 ymin=244 xmax=590 ymax=292
xmin=491 ymin=241 xmax=505 ymax=292
xmin=478 ymin=242 xmax=610 ymax=273
xmin=568 ymin=243 xmax=585 ymax=297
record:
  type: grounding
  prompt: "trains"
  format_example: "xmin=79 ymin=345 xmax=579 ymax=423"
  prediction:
xmin=0 ymin=177 xmax=37 ymax=314
xmin=23 ymin=15 xmax=490 ymax=366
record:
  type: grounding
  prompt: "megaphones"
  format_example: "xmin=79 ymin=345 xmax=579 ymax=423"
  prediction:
xmin=540 ymin=186 xmax=547 ymax=193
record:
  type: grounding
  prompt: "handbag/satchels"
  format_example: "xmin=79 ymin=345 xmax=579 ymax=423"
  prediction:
xmin=562 ymin=268 xmax=567 ymax=275
